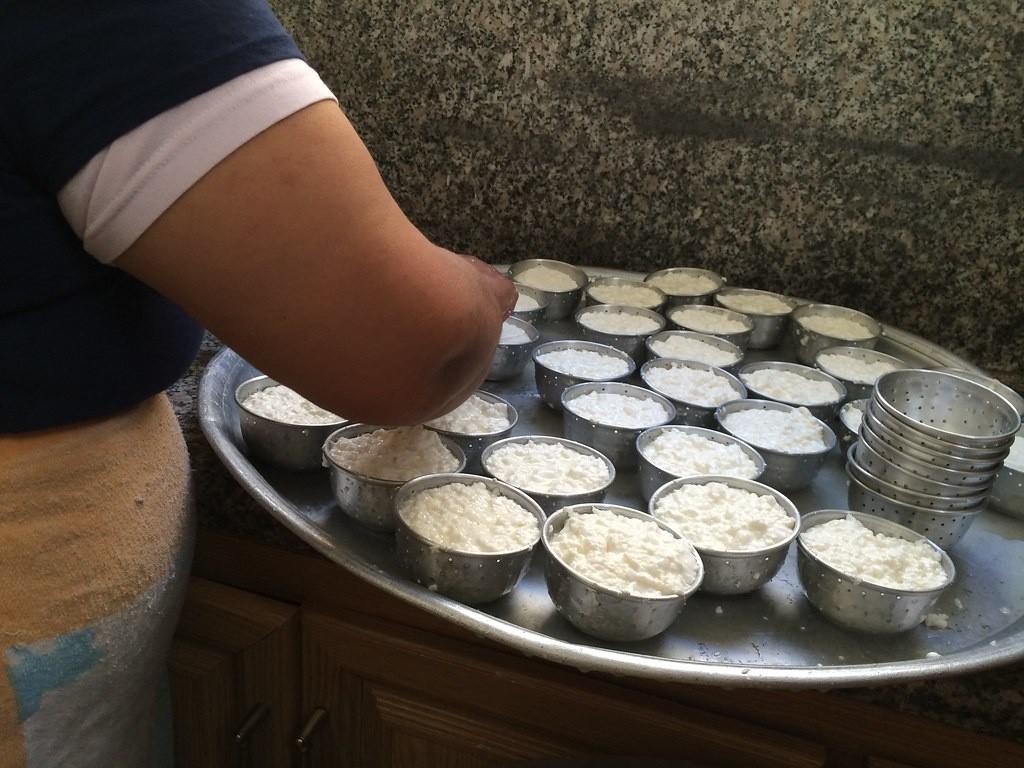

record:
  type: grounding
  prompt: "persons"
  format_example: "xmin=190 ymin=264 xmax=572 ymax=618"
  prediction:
xmin=1 ymin=3 xmax=519 ymax=767
